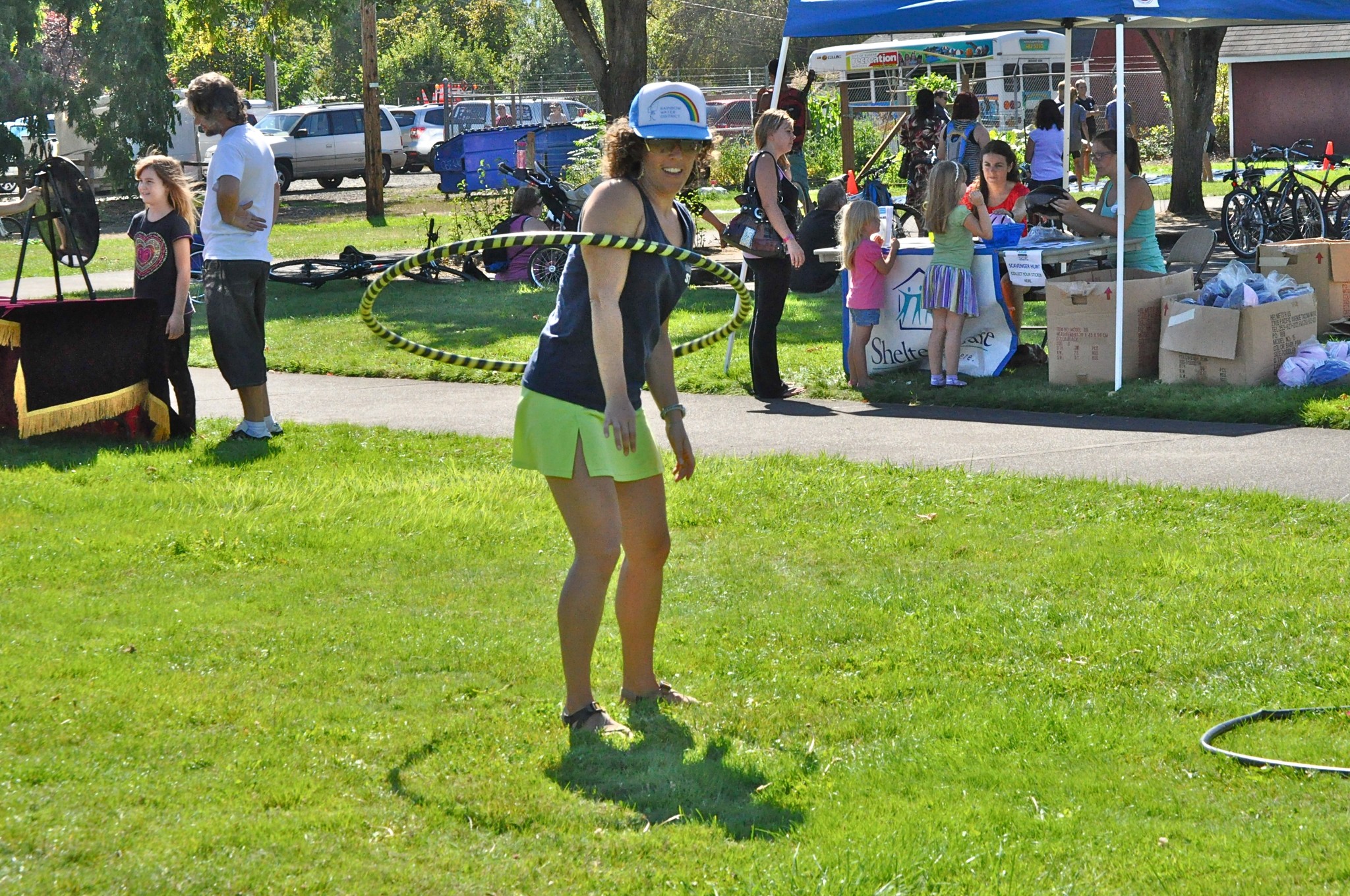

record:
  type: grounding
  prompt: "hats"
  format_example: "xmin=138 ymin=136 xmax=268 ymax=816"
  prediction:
xmin=628 ymin=81 xmax=712 ymax=140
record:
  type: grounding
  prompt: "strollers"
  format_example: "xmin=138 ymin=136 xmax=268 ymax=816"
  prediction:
xmin=496 ymin=161 xmax=593 ymax=290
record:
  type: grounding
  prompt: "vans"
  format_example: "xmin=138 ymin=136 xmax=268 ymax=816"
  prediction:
xmin=443 ymin=98 xmax=600 ymax=143
xmin=196 ymin=99 xmax=273 ymax=164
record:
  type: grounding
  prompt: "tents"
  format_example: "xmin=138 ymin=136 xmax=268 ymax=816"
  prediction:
xmin=724 ymin=0 xmax=1350 ymax=391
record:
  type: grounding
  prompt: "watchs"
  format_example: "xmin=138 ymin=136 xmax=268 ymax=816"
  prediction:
xmin=661 ymin=403 xmax=686 ymax=419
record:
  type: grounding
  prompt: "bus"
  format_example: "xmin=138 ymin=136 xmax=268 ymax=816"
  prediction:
xmin=807 ymin=30 xmax=1067 ymax=134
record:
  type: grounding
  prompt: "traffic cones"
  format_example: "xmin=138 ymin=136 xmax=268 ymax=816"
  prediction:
xmin=846 ymin=170 xmax=859 ymax=197
xmin=440 ymin=84 xmax=445 ymax=103
xmin=417 ymin=97 xmax=420 ymax=104
xmin=422 ymin=89 xmax=430 ymax=104
xmin=447 ymin=84 xmax=462 ymax=102
xmin=432 ymin=92 xmax=437 ymax=104
xmin=1321 ymin=140 xmax=1336 ymax=170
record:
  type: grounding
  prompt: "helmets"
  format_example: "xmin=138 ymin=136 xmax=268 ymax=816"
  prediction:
xmin=1025 ymin=184 xmax=1070 ymax=215
xmin=1180 ymin=274 xmax=1314 ymax=310
xmin=1277 ymin=341 xmax=1350 ymax=388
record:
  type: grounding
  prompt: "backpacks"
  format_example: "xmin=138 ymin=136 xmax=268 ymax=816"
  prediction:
xmin=863 ymin=178 xmax=892 ymax=207
xmin=766 ymin=87 xmax=806 ymax=152
xmin=481 ymin=213 xmax=533 ymax=273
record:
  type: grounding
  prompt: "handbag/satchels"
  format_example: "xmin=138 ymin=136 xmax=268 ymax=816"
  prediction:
xmin=897 ymin=152 xmax=910 ymax=180
xmin=722 ymin=152 xmax=797 ymax=260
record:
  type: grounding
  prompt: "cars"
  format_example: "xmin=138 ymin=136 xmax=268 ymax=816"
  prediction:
xmin=704 ymin=98 xmax=759 ymax=143
xmin=0 ymin=113 xmax=58 ymax=193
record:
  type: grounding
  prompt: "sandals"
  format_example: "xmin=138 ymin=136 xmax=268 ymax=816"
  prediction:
xmin=561 ymin=701 xmax=633 ymax=741
xmin=617 ymin=680 xmax=713 ymax=710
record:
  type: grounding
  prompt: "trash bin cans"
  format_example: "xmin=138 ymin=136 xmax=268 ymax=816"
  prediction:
xmin=514 ymin=122 xmax=600 ymax=187
xmin=433 ymin=123 xmax=546 ymax=200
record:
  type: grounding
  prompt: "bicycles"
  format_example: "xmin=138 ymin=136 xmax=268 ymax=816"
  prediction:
xmin=1217 ymin=138 xmax=1350 ymax=258
xmin=793 ymin=155 xmax=929 ymax=237
xmin=1020 ymin=162 xmax=1109 ymax=261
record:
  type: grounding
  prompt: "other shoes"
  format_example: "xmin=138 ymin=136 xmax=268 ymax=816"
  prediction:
xmin=928 ymin=376 xmax=945 ymax=388
xmin=946 ymin=380 xmax=969 ymax=388
xmin=226 ymin=421 xmax=273 ymax=441
xmin=783 ymin=380 xmax=793 ymax=386
xmin=268 ymin=421 xmax=283 ymax=437
xmin=782 ymin=387 xmax=805 ymax=398
xmin=720 ymin=223 xmax=730 ymax=248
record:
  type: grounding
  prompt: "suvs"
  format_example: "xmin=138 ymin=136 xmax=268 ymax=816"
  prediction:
xmin=389 ymin=102 xmax=455 ymax=174
xmin=204 ymin=102 xmax=408 ymax=197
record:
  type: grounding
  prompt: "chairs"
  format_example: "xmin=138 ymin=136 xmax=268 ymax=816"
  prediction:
xmin=314 ymin=121 xmax=327 ymax=135
xmin=1166 ymin=227 xmax=1217 ymax=291
xmin=1023 ymin=196 xmax=1107 ymax=347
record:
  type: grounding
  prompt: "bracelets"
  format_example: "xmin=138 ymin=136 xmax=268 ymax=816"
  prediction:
xmin=784 ymin=234 xmax=795 ymax=243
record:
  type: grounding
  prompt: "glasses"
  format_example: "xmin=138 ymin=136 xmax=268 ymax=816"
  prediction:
xmin=534 ymin=200 xmax=544 ymax=206
xmin=1089 ymin=151 xmax=1118 ymax=160
xmin=941 ymin=95 xmax=949 ymax=101
xmin=644 ymin=138 xmax=703 ymax=156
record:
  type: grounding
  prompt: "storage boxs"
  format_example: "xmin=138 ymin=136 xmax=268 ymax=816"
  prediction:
xmin=1044 ymin=267 xmax=1193 ymax=385
xmin=1255 ymin=237 xmax=1350 ymax=337
xmin=981 ymin=223 xmax=1025 ymax=248
xmin=1158 ymin=289 xmax=1317 ymax=386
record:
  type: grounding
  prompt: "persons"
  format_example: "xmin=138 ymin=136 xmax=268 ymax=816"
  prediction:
xmin=127 ymin=154 xmax=204 ymax=437
xmin=966 ymin=41 xmax=989 ymax=57
xmin=495 ymin=103 xmax=513 ymax=127
xmin=509 ymin=84 xmax=716 ymax=739
xmin=549 ymin=102 xmax=568 ymax=125
xmin=747 ymin=60 xmax=1167 ymax=398
xmin=0 ymin=186 xmax=43 ymax=218
xmin=897 ymin=50 xmax=922 ymax=66
xmin=186 ymin=71 xmax=283 ymax=442
xmin=491 ymin=187 xmax=555 ymax=283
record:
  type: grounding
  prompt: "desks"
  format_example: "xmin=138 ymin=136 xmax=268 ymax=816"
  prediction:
xmin=0 ymin=297 xmax=171 ymax=444
xmin=814 ymin=239 xmax=1144 ymax=377
